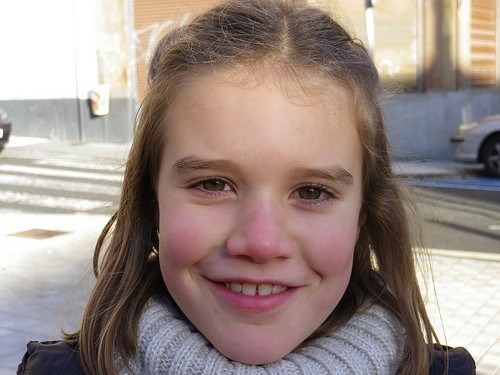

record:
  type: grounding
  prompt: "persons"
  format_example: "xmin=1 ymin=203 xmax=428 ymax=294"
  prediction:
xmin=17 ymin=1 xmax=479 ymax=375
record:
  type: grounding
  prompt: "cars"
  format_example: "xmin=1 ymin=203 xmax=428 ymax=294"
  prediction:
xmin=448 ymin=115 xmax=500 ymax=173
